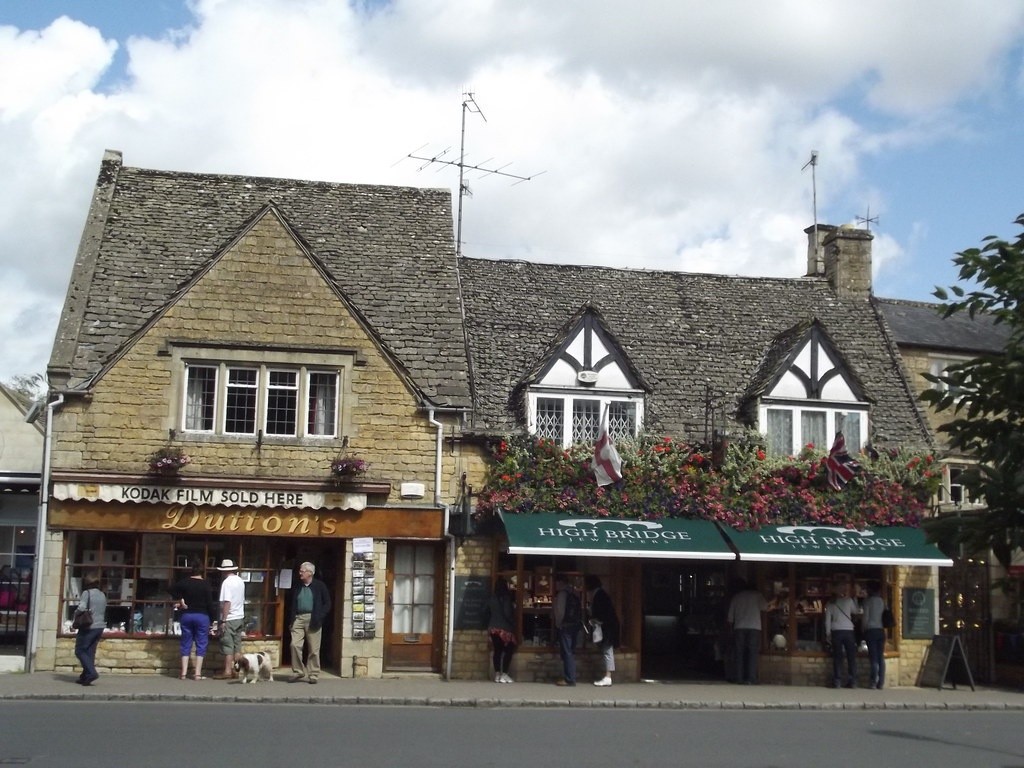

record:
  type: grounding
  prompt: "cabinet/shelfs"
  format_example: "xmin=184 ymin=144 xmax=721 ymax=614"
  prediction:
xmin=800 ymin=565 xmax=878 ymax=642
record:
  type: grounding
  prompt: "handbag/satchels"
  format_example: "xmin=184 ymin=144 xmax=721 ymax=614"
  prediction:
xmin=882 ymin=609 xmax=893 ymax=627
xmin=593 ymin=623 xmax=603 ymax=642
xmin=853 ymin=620 xmax=863 ymax=646
xmin=73 ymin=611 xmax=92 ymax=629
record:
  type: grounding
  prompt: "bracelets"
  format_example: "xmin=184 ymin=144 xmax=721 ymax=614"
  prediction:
xmin=220 ymin=620 xmax=226 ymax=623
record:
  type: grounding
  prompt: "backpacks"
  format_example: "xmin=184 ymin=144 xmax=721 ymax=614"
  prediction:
xmin=561 ymin=589 xmax=579 ymax=622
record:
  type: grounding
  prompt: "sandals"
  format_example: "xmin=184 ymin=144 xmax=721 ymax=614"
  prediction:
xmin=194 ymin=675 xmax=207 ymax=680
xmin=181 ymin=675 xmax=186 ymax=679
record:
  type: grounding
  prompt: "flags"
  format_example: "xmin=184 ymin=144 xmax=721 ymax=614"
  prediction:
xmin=590 ymin=408 xmax=622 ymax=486
xmin=826 ymin=433 xmax=860 ymax=490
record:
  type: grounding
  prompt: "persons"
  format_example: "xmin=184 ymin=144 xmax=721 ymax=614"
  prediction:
xmin=584 ymin=574 xmax=620 ymax=686
xmin=288 ymin=562 xmax=330 ymax=684
xmin=213 ymin=559 xmax=246 ymax=679
xmin=727 ymin=576 xmax=767 ymax=686
xmin=488 ymin=577 xmax=518 ymax=683
xmin=861 ymin=579 xmax=888 ymax=690
xmin=552 ymin=573 xmax=581 ymax=686
xmin=825 ymin=580 xmax=858 ymax=688
xmin=74 ymin=572 xmax=108 ymax=686
xmin=177 ymin=559 xmax=211 ymax=680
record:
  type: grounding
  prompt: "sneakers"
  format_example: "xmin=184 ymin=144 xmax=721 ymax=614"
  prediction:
xmin=500 ymin=674 xmax=515 ymax=682
xmin=494 ymin=673 xmax=500 ymax=682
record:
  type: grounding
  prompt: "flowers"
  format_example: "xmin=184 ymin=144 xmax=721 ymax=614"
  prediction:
xmin=329 ymin=455 xmax=365 ymax=489
xmin=149 ymin=447 xmax=190 ymax=472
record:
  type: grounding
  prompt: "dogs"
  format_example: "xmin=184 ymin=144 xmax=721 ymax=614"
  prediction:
xmin=233 ymin=651 xmax=273 ymax=684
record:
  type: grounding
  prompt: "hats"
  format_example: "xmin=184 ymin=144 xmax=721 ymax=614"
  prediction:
xmin=216 ymin=559 xmax=238 ymax=571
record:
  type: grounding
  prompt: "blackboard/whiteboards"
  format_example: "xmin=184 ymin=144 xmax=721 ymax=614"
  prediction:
xmin=920 ymin=634 xmax=975 ymax=688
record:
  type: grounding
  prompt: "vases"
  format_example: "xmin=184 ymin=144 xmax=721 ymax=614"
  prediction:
xmin=161 ymin=468 xmax=178 ymax=477
xmin=340 ymin=473 xmax=356 ymax=484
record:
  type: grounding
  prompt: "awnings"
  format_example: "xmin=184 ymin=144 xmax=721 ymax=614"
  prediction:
xmin=494 ymin=504 xmax=738 ymax=561
xmin=716 ymin=520 xmax=955 ymax=568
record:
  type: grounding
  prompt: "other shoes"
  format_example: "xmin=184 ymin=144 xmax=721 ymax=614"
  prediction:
xmin=309 ymin=678 xmax=316 ymax=684
xmin=594 ymin=676 xmax=612 ymax=686
xmin=213 ymin=673 xmax=233 ymax=679
xmin=77 ymin=674 xmax=85 ymax=683
xmin=557 ymin=680 xmax=575 ymax=686
xmin=84 ymin=676 xmax=98 ymax=685
xmin=288 ymin=674 xmax=304 ymax=682
